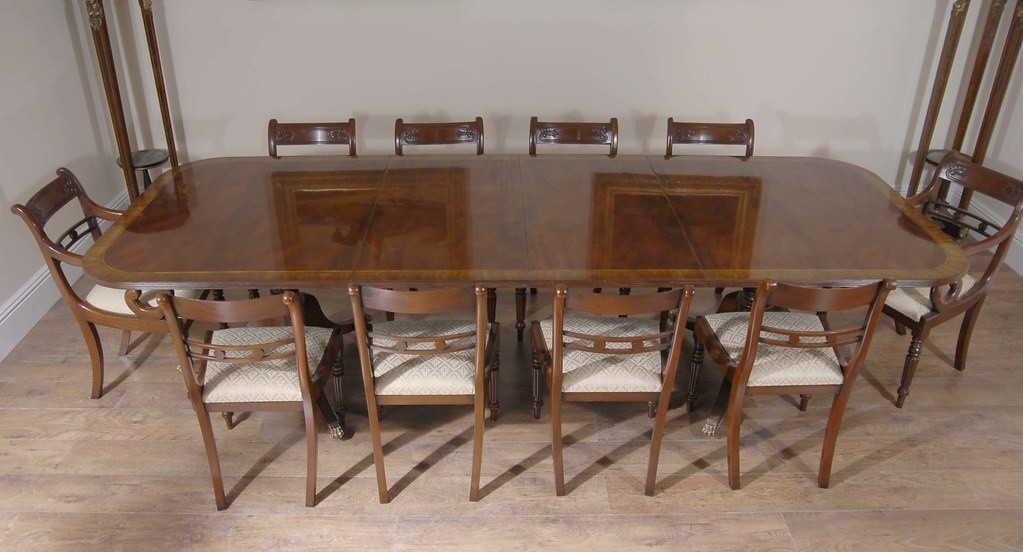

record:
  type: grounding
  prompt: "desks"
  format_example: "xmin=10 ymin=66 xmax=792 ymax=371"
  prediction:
xmin=84 ymin=156 xmax=970 ymax=333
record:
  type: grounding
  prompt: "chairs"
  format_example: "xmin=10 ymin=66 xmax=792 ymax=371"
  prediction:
xmin=156 ymin=292 xmax=343 ymax=511
xmin=687 ymin=278 xmax=898 ymax=491
xmin=267 ymin=118 xmax=356 ymax=157
xmin=531 ymin=284 xmax=694 ymax=495
xmin=348 ymin=283 xmax=500 ymax=503
xmin=882 ymin=152 xmax=1023 ymax=410
xmin=11 ymin=168 xmax=209 ymax=399
xmin=529 ymin=116 xmax=619 ymax=155
xmin=666 ymin=117 xmax=754 ymax=157
xmin=395 ymin=117 xmax=484 ymax=155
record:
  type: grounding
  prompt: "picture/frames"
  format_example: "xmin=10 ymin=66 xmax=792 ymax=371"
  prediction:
xmin=267 ymin=166 xmax=468 ymax=270
xmin=588 ymin=170 xmax=763 ymax=267
